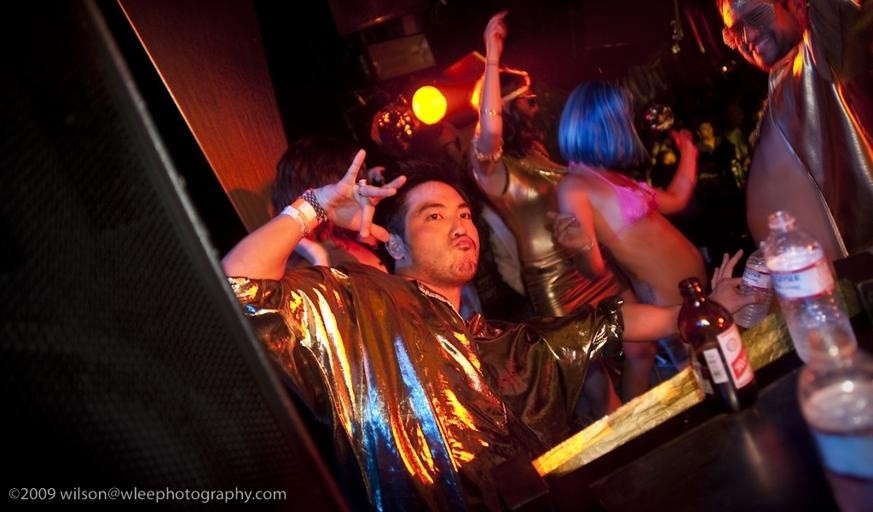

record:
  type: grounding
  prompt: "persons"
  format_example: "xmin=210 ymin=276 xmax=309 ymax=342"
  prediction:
xmin=218 ymin=0 xmax=873 ymax=512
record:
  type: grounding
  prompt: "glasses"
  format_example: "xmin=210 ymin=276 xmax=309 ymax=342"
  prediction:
xmin=721 ymin=1 xmax=777 ymax=50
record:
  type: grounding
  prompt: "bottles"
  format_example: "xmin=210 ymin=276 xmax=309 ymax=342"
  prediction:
xmin=786 ymin=321 xmax=873 ymax=511
xmin=731 ymin=239 xmax=773 ymax=329
xmin=762 ymin=210 xmax=858 ymax=364
xmin=675 ymin=276 xmax=759 ymax=414
